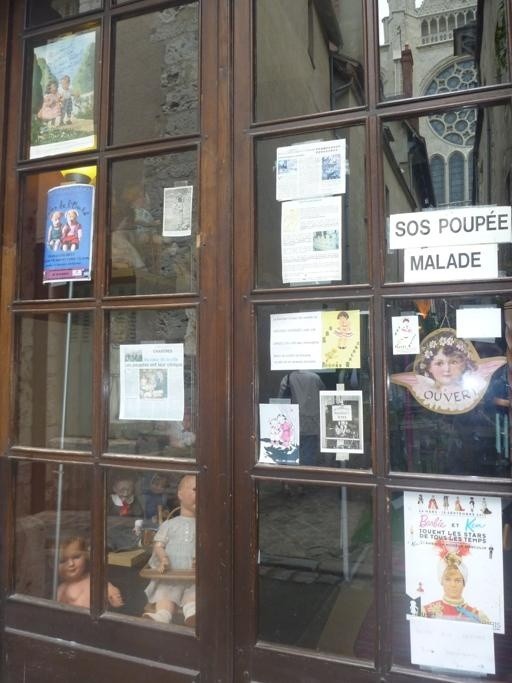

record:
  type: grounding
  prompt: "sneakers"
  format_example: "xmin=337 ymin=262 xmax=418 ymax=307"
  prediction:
xmin=184 ymin=611 xmax=197 ymax=627
xmin=143 ymin=611 xmax=170 ymax=628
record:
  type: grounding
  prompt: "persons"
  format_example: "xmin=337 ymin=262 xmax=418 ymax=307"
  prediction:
xmin=57 ymin=74 xmax=81 ymax=124
xmin=59 ymin=209 xmax=84 ymax=252
xmin=332 ymin=311 xmax=356 ymax=350
xmin=413 ymin=330 xmax=478 ymax=411
xmin=277 ymin=369 xmax=329 ymax=466
xmin=143 ymin=474 xmax=198 ymax=631
xmin=105 ymin=474 xmax=142 ymax=516
xmin=270 ymin=419 xmax=281 ymax=448
xmin=401 ymin=318 xmax=412 ymax=333
xmin=275 ymin=413 xmax=293 ymax=450
xmin=414 ymin=493 xmax=489 ymax=515
xmin=36 ymin=79 xmax=60 ymax=126
xmin=422 ymin=552 xmax=491 ymax=625
xmin=41 ymin=526 xmax=122 ymax=613
xmin=141 ymin=371 xmax=165 ymax=397
xmin=47 ymin=210 xmax=65 ymax=250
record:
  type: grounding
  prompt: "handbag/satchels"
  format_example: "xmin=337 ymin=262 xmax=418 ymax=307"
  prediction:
xmin=285 ymin=375 xmax=294 ymax=398
